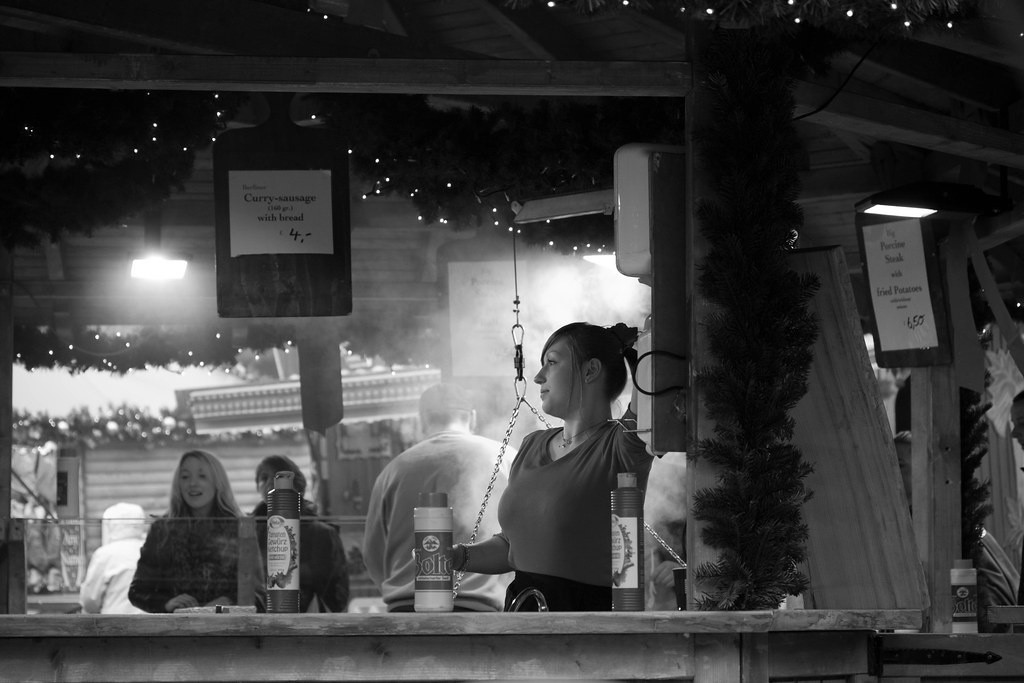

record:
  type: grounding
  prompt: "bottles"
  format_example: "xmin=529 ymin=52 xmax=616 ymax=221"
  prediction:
xmin=413 ymin=491 xmax=453 ymax=612
xmin=951 ymin=560 xmax=978 ymax=634
xmin=611 ymin=472 xmax=646 ymax=611
xmin=266 ymin=471 xmax=302 ymax=612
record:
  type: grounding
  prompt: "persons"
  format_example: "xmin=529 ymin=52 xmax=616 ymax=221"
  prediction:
xmin=1010 ymin=391 xmax=1024 ymax=451
xmin=80 ymin=503 xmax=148 ymax=614
xmin=649 ymin=560 xmax=681 ymax=588
xmin=247 ymin=455 xmax=350 ymax=613
xmin=127 ymin=450 xmax=244 ymax=613
xmin=893 ymin=430 xmax=912 ymax=518
xmin=362 ymin=384 xmax=518 ymax=613
xmin=411 ymin=322 xmax=646 ymax=614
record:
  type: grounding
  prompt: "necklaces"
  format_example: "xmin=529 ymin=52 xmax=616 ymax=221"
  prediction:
xmin=561 ymin=420 xmax=606 ymax=448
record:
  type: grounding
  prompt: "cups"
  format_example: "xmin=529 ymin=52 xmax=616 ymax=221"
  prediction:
xmin=672 ymin=567 xmax=688 ymax=610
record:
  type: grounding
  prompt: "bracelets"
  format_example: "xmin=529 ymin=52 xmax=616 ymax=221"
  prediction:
xmin=457 ymin=543 xmax=471 ymax=572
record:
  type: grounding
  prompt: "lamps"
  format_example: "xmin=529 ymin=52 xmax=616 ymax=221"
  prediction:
xmin=513 ymin=188 xmax=614 ymax=224
